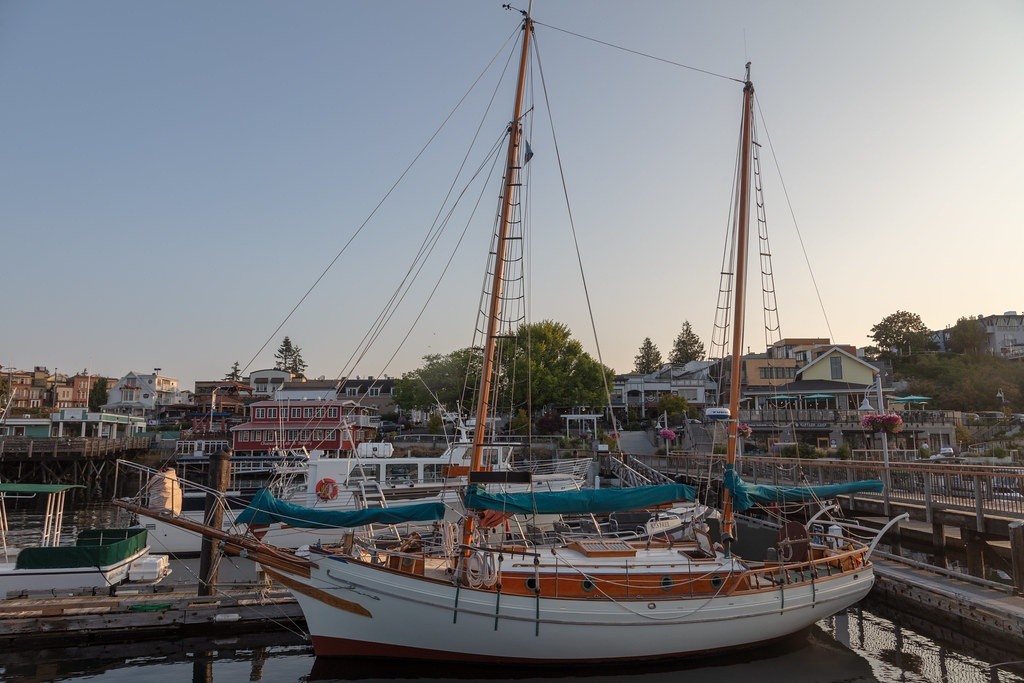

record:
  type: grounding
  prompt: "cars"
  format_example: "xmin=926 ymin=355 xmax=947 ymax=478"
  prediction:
xmin=441 ymin=412 xmax=459 ymax=425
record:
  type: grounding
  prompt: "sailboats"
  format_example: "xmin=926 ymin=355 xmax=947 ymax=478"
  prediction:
xmin=107 ymin=1 xmax=912 ymax=663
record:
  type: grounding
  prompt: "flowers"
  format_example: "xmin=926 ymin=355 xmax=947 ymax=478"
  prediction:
xmin=738 ymin=425 xmax=752 ymax=440
xmin=659 ymin=429 xmax=676 ymax=440
xmin=862 ymin=412 xmax=904 ymax=434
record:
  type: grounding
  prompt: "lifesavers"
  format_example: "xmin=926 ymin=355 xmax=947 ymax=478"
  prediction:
xmin=407 ymin=532 xmax=422 ymax=547
xmin=317 ymin=478 xmax=338 ymax=499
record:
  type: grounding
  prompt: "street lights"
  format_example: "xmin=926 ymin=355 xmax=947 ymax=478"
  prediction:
xmin=996 ymin=387 xmax=1005 ymax=410
xmin=211 ymin=386 xmax=220 ymax=432
xmin=857 ymin=374 xmax=894 ymax=491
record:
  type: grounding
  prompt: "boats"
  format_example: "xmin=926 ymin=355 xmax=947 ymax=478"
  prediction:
xmin=0 ymin=430 xmax=597 ymax=600
